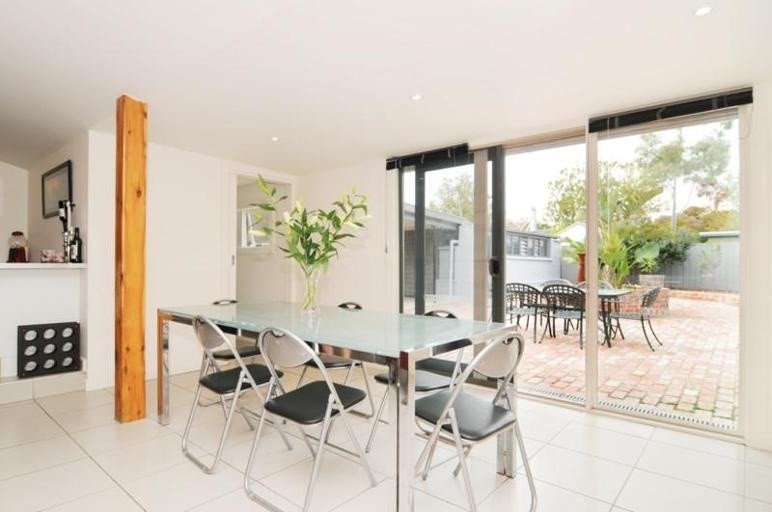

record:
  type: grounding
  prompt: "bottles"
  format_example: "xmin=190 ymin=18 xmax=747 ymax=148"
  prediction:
xmin=8 ymin=230 xmax=27 ymax=262
xmin=59 ymin=199 xmax=71 ymax=231
xmin=70 ymin=227 xmax=83 ymax=264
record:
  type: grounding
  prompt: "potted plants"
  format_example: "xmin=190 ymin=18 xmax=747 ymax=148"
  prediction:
xmin=252 ymin=174 xmax=372 ymax=354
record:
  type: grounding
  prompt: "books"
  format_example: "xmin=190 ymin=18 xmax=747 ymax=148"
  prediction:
xmin=236 ymin=208 xmax=257 ymax=248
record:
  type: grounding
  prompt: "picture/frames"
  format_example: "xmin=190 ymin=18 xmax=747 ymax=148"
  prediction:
xmin=41 ymin=159 xmax=74 ymax=219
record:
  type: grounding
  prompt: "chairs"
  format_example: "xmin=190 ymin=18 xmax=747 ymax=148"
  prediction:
xmin=497 ymin=278 xmax=661 ymax=352
xmin=243 ymin=326 xmax=374 ymax=512
xmin=363 ymin=310 xmax=466 ymax=455
xmin=416 ymin=334 xmax=539 ymax=511
xmin=198 ymin=292 xmax=258 ymax=420
xmin=181 ymin=313 xmax=291 ymax=474
xmin=297 ymin=295 xmax=379 ymax=421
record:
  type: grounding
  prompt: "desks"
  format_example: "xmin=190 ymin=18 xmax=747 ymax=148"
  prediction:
xmin=158 ymin=302 xmax=517 ymax=512
